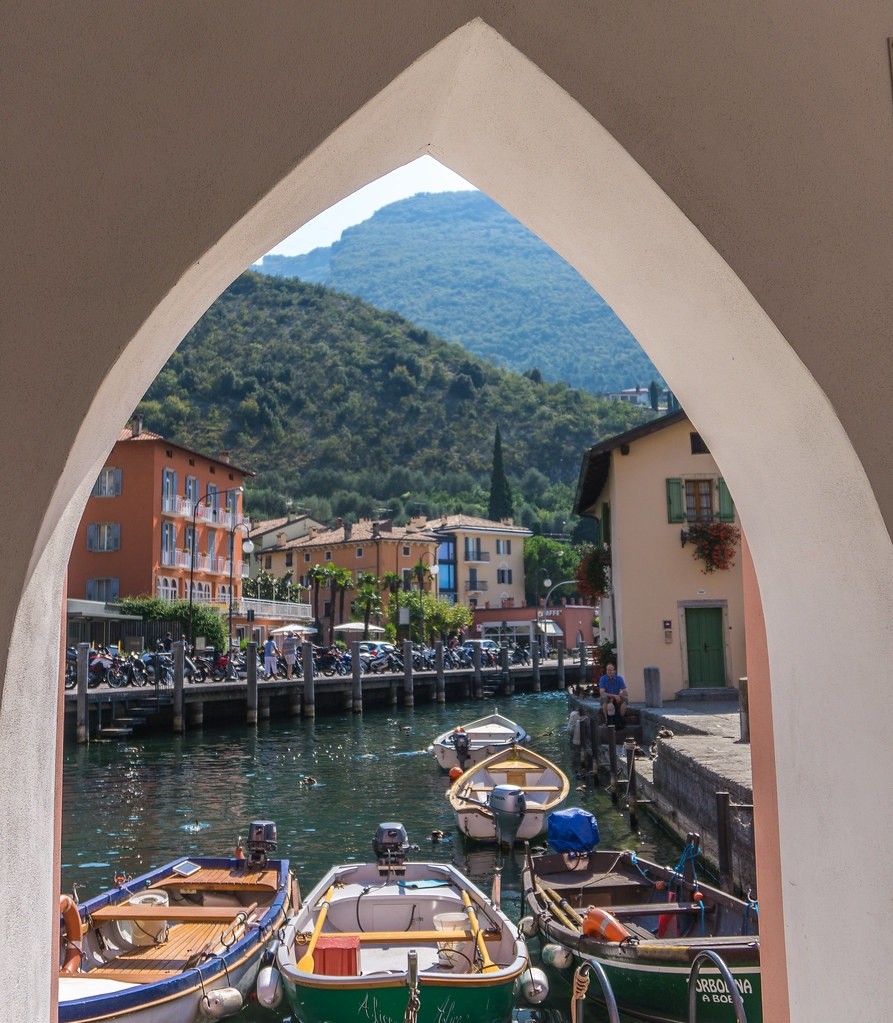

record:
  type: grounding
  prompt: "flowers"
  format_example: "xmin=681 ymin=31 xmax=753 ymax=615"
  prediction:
xmin=690 ymin=517 xmax=742 ymax=576
xmin=573 ymin=548 xmax=611 ymax=604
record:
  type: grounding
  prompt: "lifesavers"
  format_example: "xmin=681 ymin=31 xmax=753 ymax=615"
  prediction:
xmin=59 ymin=891 xmax=86 ymax=978
xmin=580 ymin=904 xmax=646 ymax=959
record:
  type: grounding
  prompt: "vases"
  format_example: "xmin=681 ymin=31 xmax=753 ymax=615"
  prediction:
xmin=599 ymin=552 xmax=611 ymax=566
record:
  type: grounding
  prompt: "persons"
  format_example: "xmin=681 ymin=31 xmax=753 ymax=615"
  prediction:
xmin=162 ymin=632 xmax=173 ymax=654
xmin=449 ymin=635 xmax=459 ymax=649
xmin=281 ymin=630 xmax=301 ymax=681
xmin=264 ymin=635 xmax=281 ymax=682
xmin=179 ymin=634 xmax=190 ymax=655
xmin=599 ymin=663 xmax=630 ymax=725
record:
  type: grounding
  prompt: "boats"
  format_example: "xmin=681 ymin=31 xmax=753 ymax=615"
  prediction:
xmin=276 ymin=822 xmax=549 ymax=1023
xmin=450 ymin=740 xmax=570 ymax=844
xmin=520 ymin=849 xmax=763 ymax=1023
xmin=431 ymin=707 xmax=530 ymax=774
xmin=57 ymin=819 xmax=297 ymax=1023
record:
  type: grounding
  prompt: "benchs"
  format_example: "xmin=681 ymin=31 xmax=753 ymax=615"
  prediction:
xmin=471 ymin=785 xmax=559 ymax=793
xmin=296 ymin=928 xmax=505 ymax=943
xmin=544 ymin=901 xmax=715 ymax=920
xmin=90 ymin=905 xmax=271 ymax=925
xmin=446 ymin=739 xmax=505 ymax=744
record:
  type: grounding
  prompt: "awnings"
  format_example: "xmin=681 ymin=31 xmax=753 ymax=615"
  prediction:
xmin=535 ymin=619 xmax=564 ymax=637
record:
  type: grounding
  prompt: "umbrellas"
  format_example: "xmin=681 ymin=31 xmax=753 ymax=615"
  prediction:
xmin=270 ymin=623 xmax=318 ymax=637
xmin=328 ymin=622 xmax=386 ymax=641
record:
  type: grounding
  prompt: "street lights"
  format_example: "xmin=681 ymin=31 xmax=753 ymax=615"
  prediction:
xmin=188 ymin=486 xmax=245 ymax=649
xmin=228 ymin=522 xmax=256 ymax=658
xmin=535 ymin=568 xmax=552 ymax=644
xmin=420 ymin=550 xmax=439 ymax=642
xmin=396 ymin=528 xmax=434 ymax=648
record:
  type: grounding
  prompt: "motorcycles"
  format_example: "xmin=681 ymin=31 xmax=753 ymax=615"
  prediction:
xmin=66 ymin=634 xmax=574 ymax=694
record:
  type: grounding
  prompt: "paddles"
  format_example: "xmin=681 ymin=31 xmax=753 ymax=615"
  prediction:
xmin=182 ymin=901 xmax=258 ymax=974
xmin=296 ymin=885 xmax=335 ymax=975
xmin=461 ymin=889 xmax=500 ymax=974
